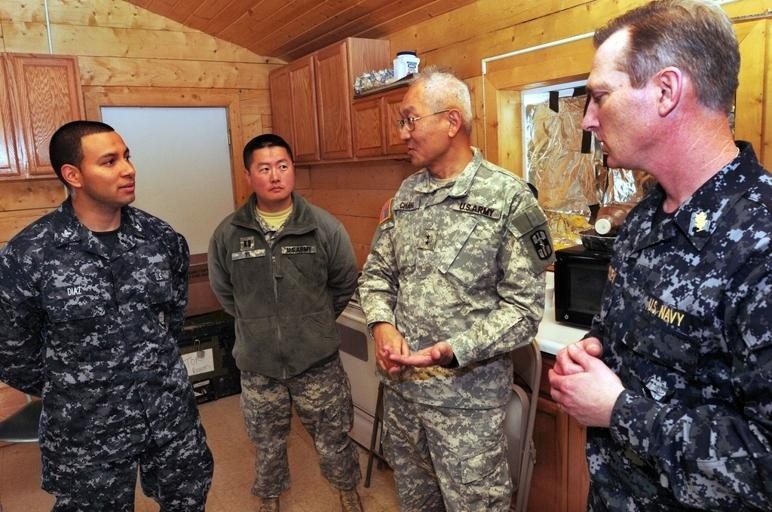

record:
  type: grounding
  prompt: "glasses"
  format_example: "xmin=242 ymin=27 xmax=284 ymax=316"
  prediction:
xmin=395 ymin=107 xmax=451 ymax=133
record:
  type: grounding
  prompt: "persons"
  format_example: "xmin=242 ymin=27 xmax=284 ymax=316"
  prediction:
xmin=0 ymin=121 xmax=214 ymax=512
xmin=209 ymin=134 xmax=363 ymax=512
xmin=357 ymin=73 xmax=556 ymax=512
xmin=549 ymin=0 xmax=772 ymax=512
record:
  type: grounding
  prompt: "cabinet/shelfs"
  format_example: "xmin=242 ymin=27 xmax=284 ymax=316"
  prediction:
xmin=0 ymin=53 xmax=86 ymax=180
xmin=530 ymin=352 xmax=590 ymax=511
xmin=261 ymin=39 xmax=416 ymax=159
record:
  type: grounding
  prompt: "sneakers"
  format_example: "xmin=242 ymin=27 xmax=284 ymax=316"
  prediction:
xmin=258 ymin=496 xmax=280 ymax=512
xmin=339 ymin=488 xmax=364 ymax=512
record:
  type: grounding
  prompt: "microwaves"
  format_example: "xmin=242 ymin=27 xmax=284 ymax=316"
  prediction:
xmin=554 ymin=246 xmax=610 ymax=330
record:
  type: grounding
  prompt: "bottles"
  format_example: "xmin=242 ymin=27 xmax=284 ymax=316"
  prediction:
xmin=594 ymin=202 xmax=634 ymax=235
xmin=393 ymin=51 xmax=420 ymax=81
